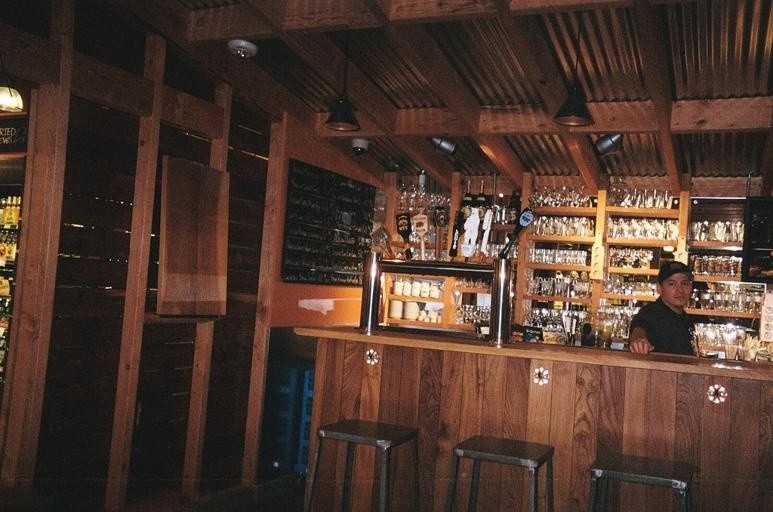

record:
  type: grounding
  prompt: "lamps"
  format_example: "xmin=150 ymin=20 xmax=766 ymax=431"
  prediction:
xmin=430 ymin=136 xmax=458 ymax=154
xmin=227 ymin=39 xmax=258 ymax=59
xmin=594 ymin=132 xmax=623 ymax=154
xmin=553 ymin=10 xmax=596 ymax=127
xmin=0 ymin=62 xmax=24 ymax=112
xmin=323 ymin=31 xmax=361 ymax=133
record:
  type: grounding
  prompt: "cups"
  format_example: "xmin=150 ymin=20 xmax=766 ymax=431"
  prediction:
xmin=530 ymin=248 xmax=587 ymax=264
xmin=529 ymin=276 xmax=578 ymax=299
xmin=597 ymin=305 xmax=615 ymax=348
xmin=462 ymin=304 xmax=490 ymax=321
xmin=537 ymin=215 xmax=594 ymax=237
xmin=580 ymin=307 xmax=596 ymax=346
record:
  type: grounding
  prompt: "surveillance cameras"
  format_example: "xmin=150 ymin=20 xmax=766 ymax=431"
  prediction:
xmin=350 ymin=138 xmax=370 ymax=158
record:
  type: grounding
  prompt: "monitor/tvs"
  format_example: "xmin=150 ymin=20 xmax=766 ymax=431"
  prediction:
xmin=742 ymin=196 xmax=773 ymax=284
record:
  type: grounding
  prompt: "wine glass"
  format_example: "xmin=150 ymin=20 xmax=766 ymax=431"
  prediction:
xmin=540 ymin=183 xmax=589 ymax=207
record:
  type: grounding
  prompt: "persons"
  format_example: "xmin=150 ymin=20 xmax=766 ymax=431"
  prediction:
xmin=625 ymin=260 xmax=698 ymax=358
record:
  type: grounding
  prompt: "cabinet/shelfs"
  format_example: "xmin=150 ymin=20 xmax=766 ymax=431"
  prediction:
xmin=0 ymin=183 xmax=23 ymax=385
xmin=371 ymin=173 xmax=773 ymax=365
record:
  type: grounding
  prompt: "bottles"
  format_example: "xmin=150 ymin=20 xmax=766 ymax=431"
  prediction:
xmin=664 ymin=189 xmax=669 ymax=208
xmin=412 ymin=215 xmax=428 ymax=260
xmin=632 ymin=187 xmax=638 ymax=204
xmin=495 ymin=192 xmax=520 ymax=224
xmin=462 ymin=178 xmax=488 ymax=218
xmin=399 ymin=170 xmax=427 ymax=210
xmin=690 ymin=253 xmax=741 ymax=276
xmin=653 ymin=188 xmax=658 ymax=205
xmin=396 ymin=213 xmax=412 ymax=261
xmin=644 ymin=188 xmax=648 ymax=206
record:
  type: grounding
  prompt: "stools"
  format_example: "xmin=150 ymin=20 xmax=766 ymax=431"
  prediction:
xmin=306 ymin=419 xmax=697 ymax=512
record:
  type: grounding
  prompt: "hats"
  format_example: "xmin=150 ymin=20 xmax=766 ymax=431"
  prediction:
xmin=658 ymin=261 xmax=694 ymax=283
